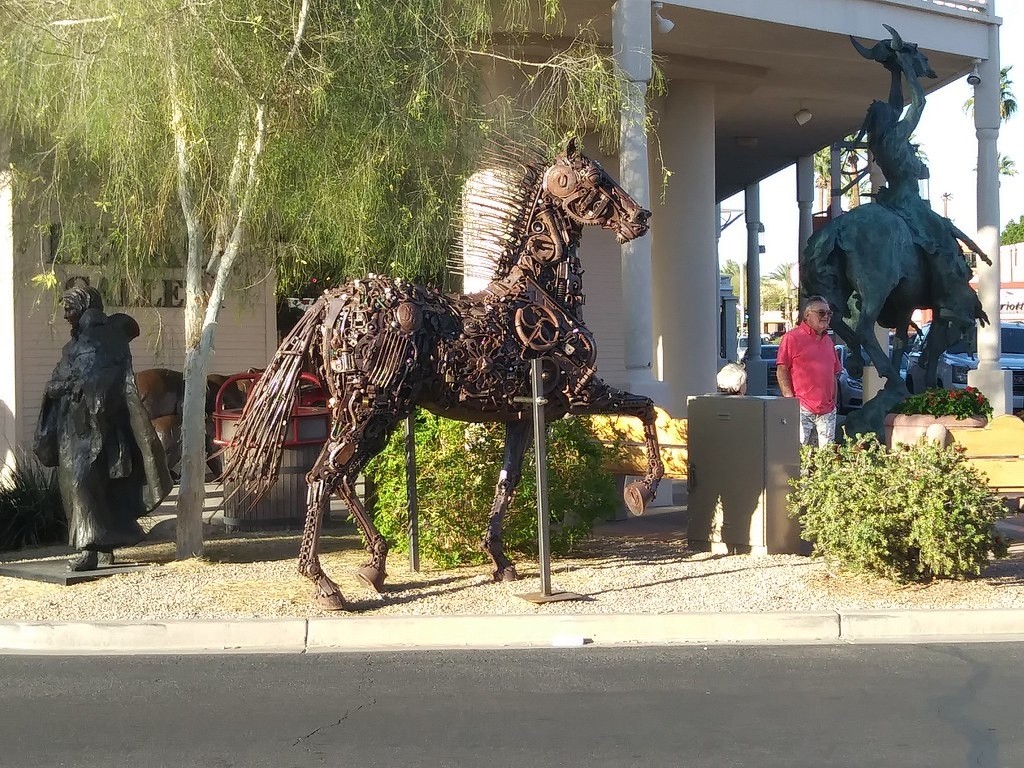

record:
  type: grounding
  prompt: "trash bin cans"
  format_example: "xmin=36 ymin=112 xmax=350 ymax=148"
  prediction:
xmin=212 ymin=372 xmax=332 ymax=534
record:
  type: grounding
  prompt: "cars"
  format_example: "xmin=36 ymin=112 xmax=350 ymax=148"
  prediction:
xmin=733 ymin=329 xmax=919 ymax=411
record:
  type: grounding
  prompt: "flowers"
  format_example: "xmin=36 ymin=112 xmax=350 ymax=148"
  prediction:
xmin=883 ymin=385 xmax=995 ymax=421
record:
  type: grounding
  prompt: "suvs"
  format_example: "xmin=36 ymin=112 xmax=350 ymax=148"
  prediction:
xmin=907 ymin=319 xmax=1024 ymax=414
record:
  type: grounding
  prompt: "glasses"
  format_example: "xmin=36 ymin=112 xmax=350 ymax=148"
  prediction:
xmin=810 ymin=309 xmax=833 ymax=317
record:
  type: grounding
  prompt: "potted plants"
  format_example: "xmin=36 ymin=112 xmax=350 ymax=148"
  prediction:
xmin=883 ymin=414 xmax=988 ymax=452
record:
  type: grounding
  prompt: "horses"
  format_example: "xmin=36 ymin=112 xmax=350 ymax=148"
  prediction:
xmin=210 ymin=137 xmax=664 ymax=608
xmin=797 ymin=203 xmax=978 ymax=392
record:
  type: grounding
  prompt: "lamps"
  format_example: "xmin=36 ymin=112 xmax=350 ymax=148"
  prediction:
xmin=968 ymin=59 xmax=982 ymax=85
xmin=793 ymin=98 xmax=813 ymax=127
xmin=652 ymin=1 xmax=675 ymax=34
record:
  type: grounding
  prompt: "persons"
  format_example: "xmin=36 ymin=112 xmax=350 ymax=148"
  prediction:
xmin=865 ymin=52 xmax=983 ymax=322
xmin=777 ymin=295 xmax=844 ymax=454
xmin=34 ymin=287 xmax=175 ymax=572
